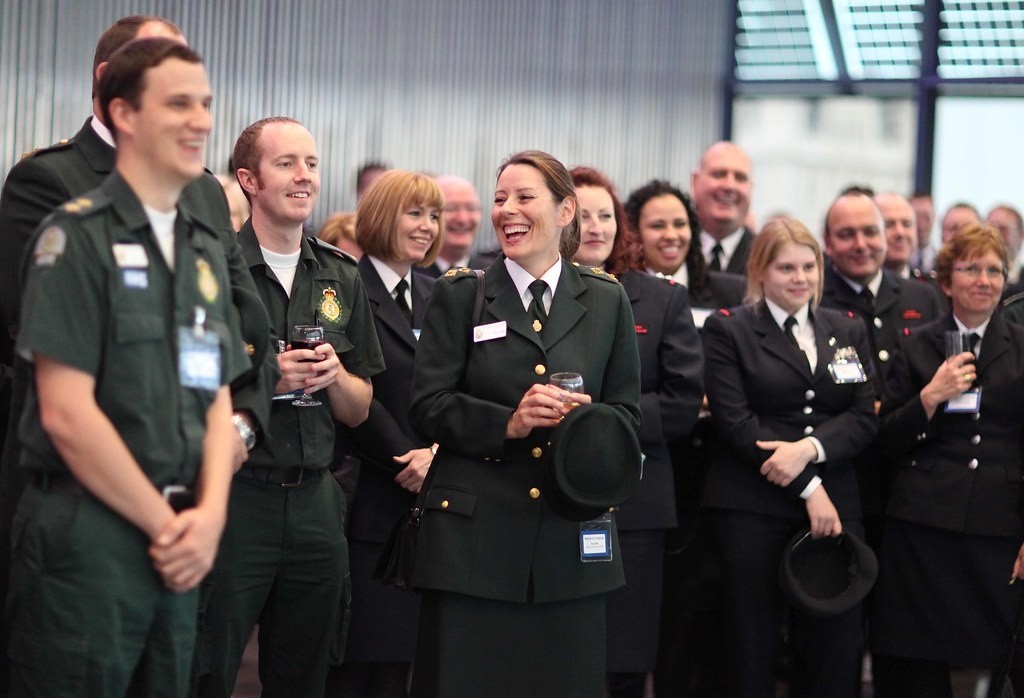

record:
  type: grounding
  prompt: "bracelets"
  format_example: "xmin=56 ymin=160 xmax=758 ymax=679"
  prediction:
xmin=431 ymin=447 xmax=437 ymax=454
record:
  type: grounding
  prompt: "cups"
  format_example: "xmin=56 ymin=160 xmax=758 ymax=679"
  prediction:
xmin=550 ymin=373 xmax=584 ymax=421
xmin=944 ymin=331 xmax=971 ymax=369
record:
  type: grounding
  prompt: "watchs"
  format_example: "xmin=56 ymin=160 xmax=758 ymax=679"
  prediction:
xmin=232 ymin=414 xmax=255 ymax=449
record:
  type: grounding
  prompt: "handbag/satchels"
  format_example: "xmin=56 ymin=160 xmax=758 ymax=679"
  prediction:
xmin=383 ymin=517 xmax=419 ymax=592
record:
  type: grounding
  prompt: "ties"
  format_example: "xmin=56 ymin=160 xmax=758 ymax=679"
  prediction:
xmin=526 ymin=279 xmax=547 ymax=338
xmin=709 ymin=244 xmax=723 ymax=274
xmin=962 ymin=333 xmax=981 ymax=364
xmin=858 ymin=287 xmax=873 ymax=313
xmin=393 ymin=279 xmax=410 ymax=312
xmin=783 ymin=316 xmax=811 ymax=377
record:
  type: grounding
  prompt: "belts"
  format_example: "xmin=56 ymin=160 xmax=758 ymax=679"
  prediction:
xmin=244 ymin=464 xmax=327 ymax=485
xmin=153 ymin=486 xmax=199 ymax=514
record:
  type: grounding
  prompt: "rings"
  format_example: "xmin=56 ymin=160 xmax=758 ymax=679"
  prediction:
xmin=965 ymin=374 xmax=971 ymax=381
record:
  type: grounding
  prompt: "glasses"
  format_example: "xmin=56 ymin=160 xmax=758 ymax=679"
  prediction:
xmin=951 ymin=264 xmax=1003 ymax=276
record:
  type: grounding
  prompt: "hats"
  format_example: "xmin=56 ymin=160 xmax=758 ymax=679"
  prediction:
xmin=786 ymin=523 xmax=879 ymax=614
xmin=551 ymin=404 xmax=643 ymax=508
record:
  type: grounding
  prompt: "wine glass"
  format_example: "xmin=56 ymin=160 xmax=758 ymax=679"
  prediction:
xmin=291 ymin=325 xmax=324 ymax=407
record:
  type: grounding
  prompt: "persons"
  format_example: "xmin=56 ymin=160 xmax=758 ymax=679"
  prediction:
xmin=0 ymin=37 xmax=252 ymax=698
xmin=0 ymin=13 xmax=284 ymax=524
xmin=194 ymin=115 xmax=1024 ymax=698
